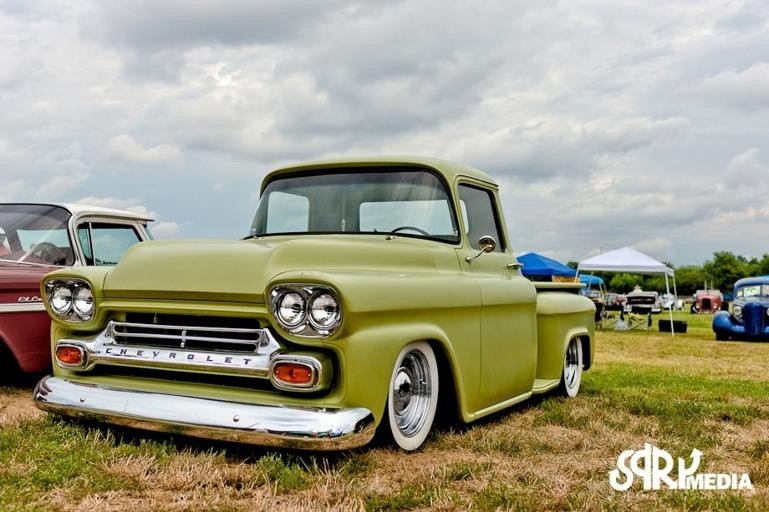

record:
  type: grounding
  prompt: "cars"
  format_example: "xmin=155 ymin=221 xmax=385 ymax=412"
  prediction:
xmin=690 ymin=275 xmax=769 ymax=341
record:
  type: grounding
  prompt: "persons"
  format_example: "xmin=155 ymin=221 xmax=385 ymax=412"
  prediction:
xmin=0 ymin=227 xmax=11 ymax=256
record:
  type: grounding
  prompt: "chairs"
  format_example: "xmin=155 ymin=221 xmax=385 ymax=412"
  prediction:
xmin=594 ymin=302 xmax=652 ymax=332
